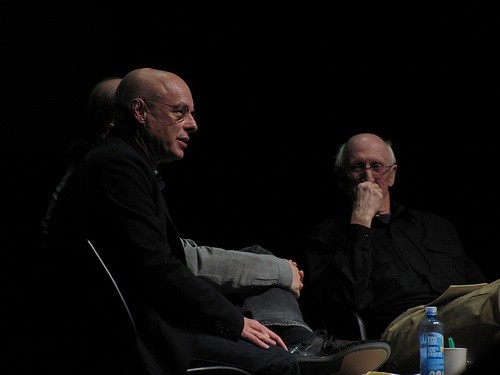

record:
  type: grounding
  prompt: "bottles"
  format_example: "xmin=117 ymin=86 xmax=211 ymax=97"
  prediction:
xmin=419 ymin=306 xmax=446 ymax=375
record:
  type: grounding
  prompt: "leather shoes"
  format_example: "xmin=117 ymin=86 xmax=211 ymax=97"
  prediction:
xmin=286 ymin=329 xmax=391 ymax=375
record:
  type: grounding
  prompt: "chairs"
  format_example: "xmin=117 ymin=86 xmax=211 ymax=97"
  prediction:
xmin=79 ymin=236 xmax=251 ymax=375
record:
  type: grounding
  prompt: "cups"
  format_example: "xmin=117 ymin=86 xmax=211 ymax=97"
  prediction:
xmin=444 ymin=347 xmax=469 ymax=375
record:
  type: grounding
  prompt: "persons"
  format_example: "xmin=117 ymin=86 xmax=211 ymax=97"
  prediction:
xmin=300 ymin=132 xmax=500 ymax=375
xmin=46 ymin=69 xmax=300 ymax=374
xmin=48 ymin=78 xmax=392 ymax=375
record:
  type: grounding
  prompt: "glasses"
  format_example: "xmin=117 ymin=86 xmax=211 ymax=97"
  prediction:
xmin=343 ymin=163 xmax=395 ymax=178
xmin=143 ymin=97 xmax=197 ymax=121
